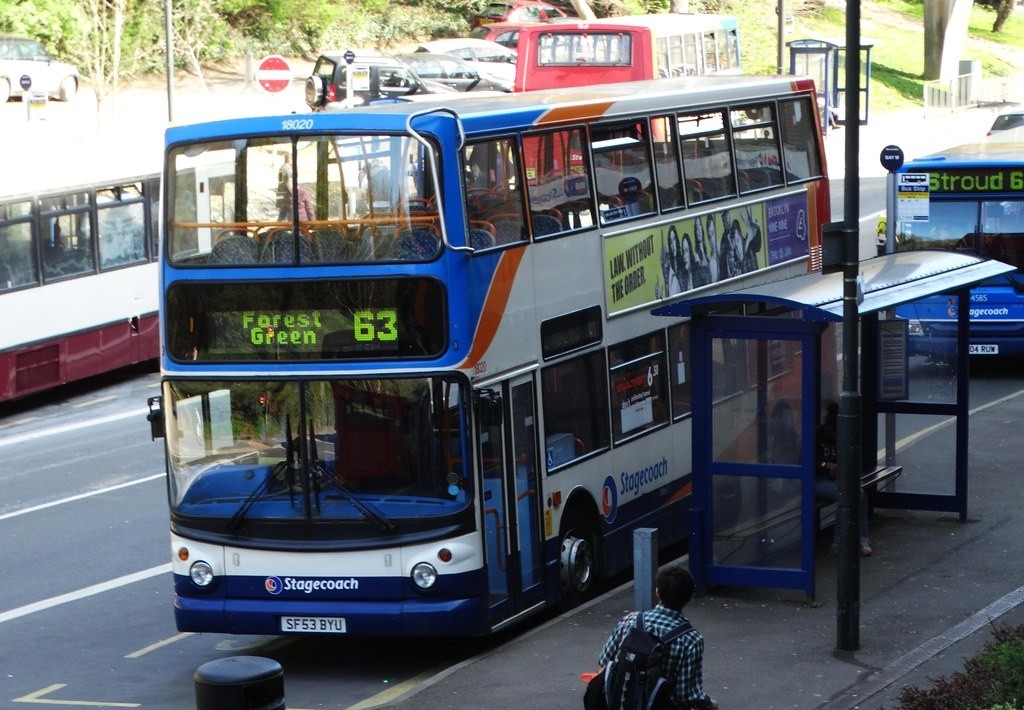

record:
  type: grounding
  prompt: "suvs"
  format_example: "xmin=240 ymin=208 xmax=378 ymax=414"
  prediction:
xmin=0 ymin=35 xmax=82 ymax=105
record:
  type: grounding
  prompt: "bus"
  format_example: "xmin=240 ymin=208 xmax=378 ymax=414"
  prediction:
xmin=143 ymin=72 xmax=833 ymax=643
xmin=873 ymin=124 xmax=1024 ymax=358
xmin=514 ymin=10 xmax=745 ymax=186
xmin=0 ymin=158 xmax=235 ymax=408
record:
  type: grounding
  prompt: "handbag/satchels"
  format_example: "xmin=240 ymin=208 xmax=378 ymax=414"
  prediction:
xmin=584 ymin=669 xmax=606 ymax=710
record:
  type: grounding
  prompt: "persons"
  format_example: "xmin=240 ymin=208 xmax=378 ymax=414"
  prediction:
xmin=495 ymin=148 xmax=515 ymax=192
xmin=655 ymin=206 xmax=762 ymax=299
xmin=369 ymin=159 xmax=399 ymax=207
xmin=816 ymin=397 xmax=874 ymax=555
xmin=277 ymin=170 xmax=315 ymax=221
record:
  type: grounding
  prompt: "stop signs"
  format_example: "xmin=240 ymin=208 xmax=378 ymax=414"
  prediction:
xmin=256 ymin=54 xmax=292 ymax=93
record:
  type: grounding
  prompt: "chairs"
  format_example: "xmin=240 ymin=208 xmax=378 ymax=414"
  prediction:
xmin=207 ymin=153 xmax=781 ymax=265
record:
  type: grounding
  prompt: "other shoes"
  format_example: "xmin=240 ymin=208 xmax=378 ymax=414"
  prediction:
xmin=832 ymin=543 xmax=840 ymax=555
xmin=860 ymin=537 xmax=873 ymax=555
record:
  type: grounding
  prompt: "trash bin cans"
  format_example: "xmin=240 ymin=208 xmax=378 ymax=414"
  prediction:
xmin=194 ymin=654 xmax=287 ymax=710
xmin=959 ymin=60 xmax=982 ymax=100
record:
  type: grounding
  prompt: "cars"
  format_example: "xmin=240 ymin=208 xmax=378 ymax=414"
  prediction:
xmin=985 ymin=110 xmax=1024 ymax=136
xmin=302 ymin=0 xmax=571 ymax=111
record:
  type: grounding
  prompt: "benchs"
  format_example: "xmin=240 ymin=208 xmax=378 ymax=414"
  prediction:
xmin=713 ymin=464 xmax=904 ymax=564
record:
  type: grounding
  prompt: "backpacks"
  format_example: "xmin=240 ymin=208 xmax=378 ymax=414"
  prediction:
xmin=604 ymin=610 xmax=695 ymax=710
